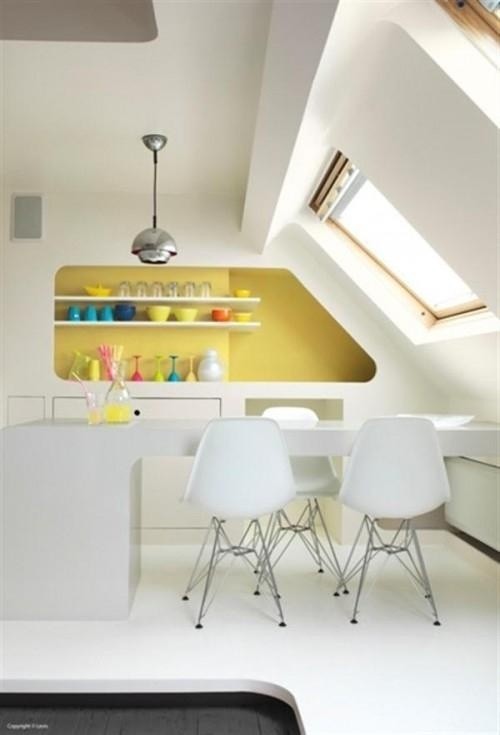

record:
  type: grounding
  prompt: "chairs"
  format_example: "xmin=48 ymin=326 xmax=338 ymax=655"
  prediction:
xmin=252 ymin=407 xmax=349 ymax=598
xmin=183 ymin=417 xmax=293 ymax=628
xmin=334 ymin=415 xmax=451 ymax=624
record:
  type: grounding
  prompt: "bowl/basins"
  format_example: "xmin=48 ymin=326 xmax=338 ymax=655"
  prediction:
xmin=212 ymin=288 xmax=254 ymax=321
xmin=113 ymin=305 xmax=197 ymax=321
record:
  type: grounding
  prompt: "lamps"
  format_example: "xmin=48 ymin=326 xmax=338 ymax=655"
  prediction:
xmin=132 ymin=134 xmax=178 ymax=264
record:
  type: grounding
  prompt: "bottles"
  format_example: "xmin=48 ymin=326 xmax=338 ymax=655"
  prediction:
xmin=103 ymin=361 xmax=135 ymax=422
xmin=199 ymin=349 xmax=222 ymax=381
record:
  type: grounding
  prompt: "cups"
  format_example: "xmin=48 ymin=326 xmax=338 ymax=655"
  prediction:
xmin=84 ymin=392 xmax=105 ymax=424
xmin=88 ymin=358 xmax=100 ymax=378
xmin=117 ymin=281 xmax=210 ymax=300
xmin=65 ymin=305 xmax=113 ymax=321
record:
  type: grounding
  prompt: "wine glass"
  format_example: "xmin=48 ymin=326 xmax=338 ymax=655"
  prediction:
xmin=133 ymin=355 xmax=196 ymax=381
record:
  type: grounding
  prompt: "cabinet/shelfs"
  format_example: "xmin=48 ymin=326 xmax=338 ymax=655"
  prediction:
xmin=54 ymin=296 xmax=261 ymax=332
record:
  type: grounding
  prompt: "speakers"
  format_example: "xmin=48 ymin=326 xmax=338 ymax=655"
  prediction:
xmin=11 ymin=191 xmax=48 ymax=243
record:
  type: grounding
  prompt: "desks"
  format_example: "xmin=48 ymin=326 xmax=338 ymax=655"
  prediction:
xmin=2 ymin=418 xmax=499 ymax=620
